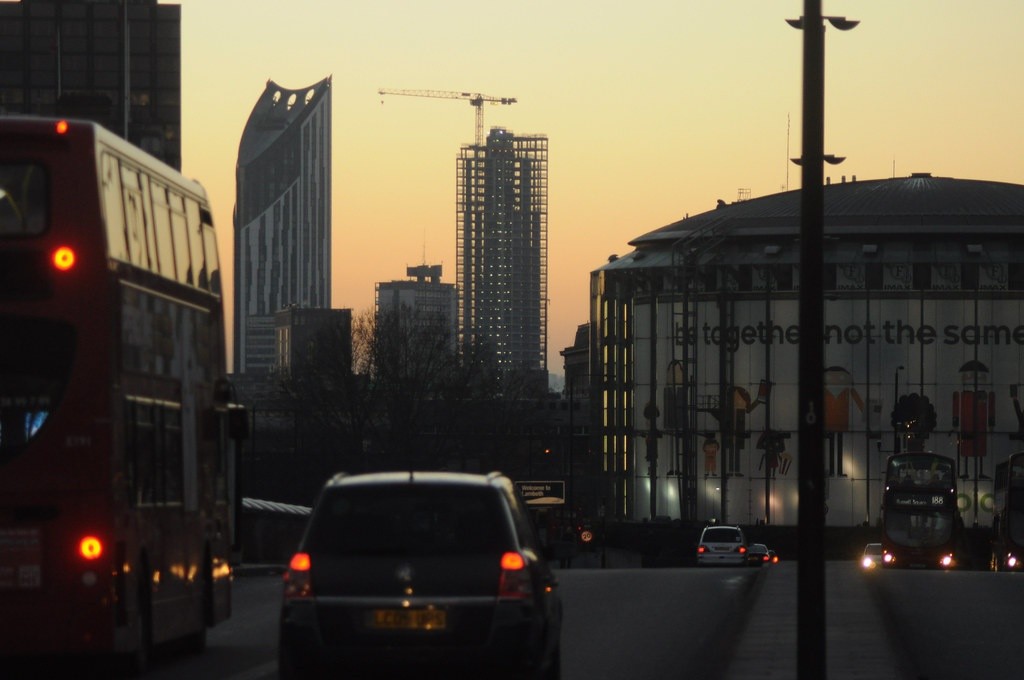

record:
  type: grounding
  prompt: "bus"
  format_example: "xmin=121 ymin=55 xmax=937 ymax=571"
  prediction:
xmin=993 ymin=453 xmax=1024 ymax=573
xmin=882 ymin=453 xmax=958 ymax=570
xmin=0 ymin=116 xmax=249 ymax=680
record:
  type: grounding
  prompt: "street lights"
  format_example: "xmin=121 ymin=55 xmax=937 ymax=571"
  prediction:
xmin=894 ymin=365 xmax=905 ymax=453
xmin=567 ymin=374 xmax=615 ymax=570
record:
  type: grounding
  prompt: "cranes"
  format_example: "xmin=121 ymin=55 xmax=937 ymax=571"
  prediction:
xmin=379 ymin=87 xmax=517 ymax=213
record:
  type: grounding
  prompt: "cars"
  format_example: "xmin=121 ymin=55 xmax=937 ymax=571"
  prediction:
xmin=697 ymin=525 xmax=749 ymax=566
xmin=276 ymin=470 xmax=562 ymax=680
xmin=862 ymin=544 xmax=882 ymax=569
xmin=747 ymin=543 xmax=779 ymax=567
